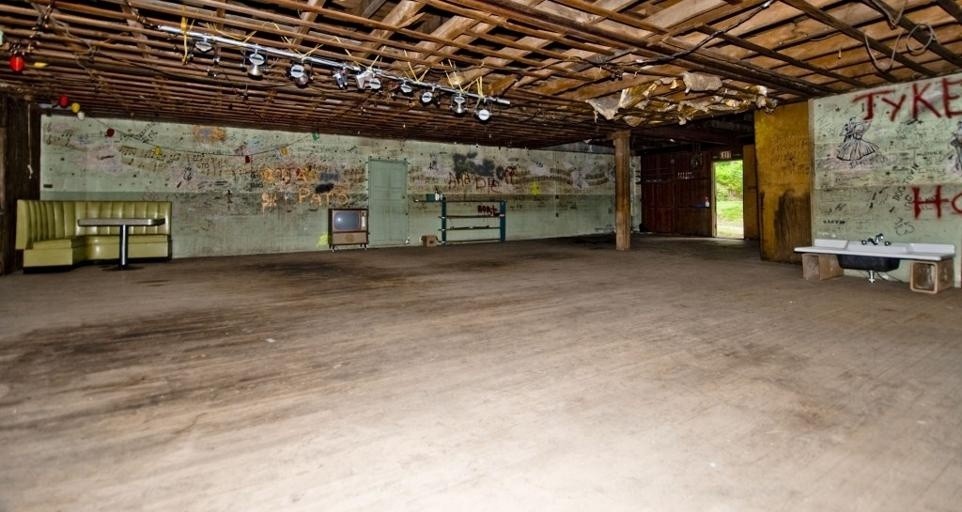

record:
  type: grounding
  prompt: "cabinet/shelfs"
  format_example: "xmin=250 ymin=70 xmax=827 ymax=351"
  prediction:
xmin=442 ymin=195 xmax=506 ymax=245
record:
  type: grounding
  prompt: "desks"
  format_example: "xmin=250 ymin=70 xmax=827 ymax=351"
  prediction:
xmin=77 ymin=212 xmax=165 ymax=271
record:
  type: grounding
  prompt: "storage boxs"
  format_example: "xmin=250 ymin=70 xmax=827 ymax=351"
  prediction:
xmin=421 ymin=235 xmax=437 ymax=247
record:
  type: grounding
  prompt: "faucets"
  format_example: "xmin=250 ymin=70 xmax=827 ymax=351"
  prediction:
xmin=861 ymin=233 xmax=884 ymax=247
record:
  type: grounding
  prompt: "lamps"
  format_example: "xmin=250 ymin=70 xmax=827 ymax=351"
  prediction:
xmin=161 ymin=26 xmax=511 ymax=123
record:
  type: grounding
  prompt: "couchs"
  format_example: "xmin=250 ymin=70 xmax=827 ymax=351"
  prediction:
xmin=17 ymin=198 xmax=173 ymax=269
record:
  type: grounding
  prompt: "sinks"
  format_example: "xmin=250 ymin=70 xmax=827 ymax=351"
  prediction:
xmin=843 ymin=240 xmax=909 ymax=258
